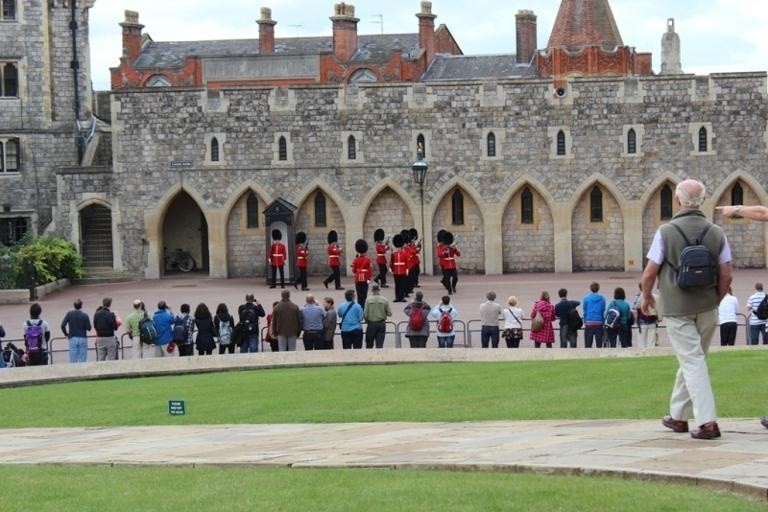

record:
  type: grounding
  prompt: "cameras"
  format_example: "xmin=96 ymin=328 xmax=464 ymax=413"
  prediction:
xmin=253 ymin=298 xmax=256 ymax=302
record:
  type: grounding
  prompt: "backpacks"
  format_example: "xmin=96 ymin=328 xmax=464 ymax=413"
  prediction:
xmin=410 ymin=305 xmax=424 ymax=330
xmin=173 ymin=315 xmax=190 ymax=343
xmin=664 ymin=222 xmax=713 ymax=288
xmin=26 ymin=320 xmax=42 ymax=351
xmin=438 ymin=308 xmax=453 ymax=332
xmin=218 ymin=317 xmax=233 ymax=345
xmin=241 ymin=305 xmax=257 ymax=334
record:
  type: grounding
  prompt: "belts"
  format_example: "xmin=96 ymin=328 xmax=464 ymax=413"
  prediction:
xmin=393 ymin=262 xmax=405 ymax=265
xmin=444 ymin=257 xmax=455 ymax=260
xmin=377 ymin=254 xmax=386 ymax=256
xmin=272 ymin=254 xmax=283 ymax=256
xmin=297 ymin=257 xmax=307 ymax=259
xmin=329 ymin=255 xmax=339 ymax=257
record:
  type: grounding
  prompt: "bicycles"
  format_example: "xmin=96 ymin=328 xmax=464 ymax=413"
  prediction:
xmin=163 ymin=247 xmax=196 ymax=273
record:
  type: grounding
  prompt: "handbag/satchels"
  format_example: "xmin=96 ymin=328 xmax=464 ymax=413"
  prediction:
xmin=604 ymin=306 xmax=621 ymax=329
xmin=139 ymin=318 xmax=158 ymax=342
xmin=757 ymin=294 xmax=768 ymax=320
xmin=569 ymin=309 xmax=582 ymax=330
xmin=532 ymin=312 xmax=544 ymax=330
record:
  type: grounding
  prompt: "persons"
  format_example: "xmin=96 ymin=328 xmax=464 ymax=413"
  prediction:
xmin=0 ymin=282 xmax=768 ymax=366
xmin=640 ymin=179 xmax=734 ymax=438
xmin=267 ymin=229 xmax=461 ymax=302
xmin=714 ymin=205 xmax=768 ymax=428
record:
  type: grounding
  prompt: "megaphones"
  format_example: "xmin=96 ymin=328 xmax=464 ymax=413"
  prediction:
xmin=557 ymin=87 xmax=567 ymax=97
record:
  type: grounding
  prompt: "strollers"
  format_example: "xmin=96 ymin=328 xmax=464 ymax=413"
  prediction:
xmin=4 ymin=342 xmax=29 ymax=367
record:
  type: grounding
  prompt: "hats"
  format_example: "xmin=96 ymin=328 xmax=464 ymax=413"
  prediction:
xmin=355 ymin=239 xmax=367 ymax=253
xmin=392 ymin=228 xmax=418 ymax=246
xmin=295 ymin=232 xmax=306 ymax=244
xmin=272 ymin=229 xmax=281 ymax=240
xmin=437 ymin=230 xmax=453 ymax=244
xmin=328 ymin=230 xmax=337 ymax=243
xmin=375 ymin=229 xmax=384 ymax=241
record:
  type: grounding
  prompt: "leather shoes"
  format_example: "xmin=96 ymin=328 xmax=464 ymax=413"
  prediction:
xmin=381 ymin=284 xmax=389 ymax=288
xmin=692 ymin=423 xmax=720 ymax=438
xmin=292 ymin=280 xmax=298 ymax=289
xmin=441 ymin=280 xmax=456 ymax=295
xmin=302 ymin=287 xmax=310 ymax=290
xmin=393 ymin=285 xmax=420 ymax=302
xmin=270 ymin=284 xmax=275 ymax=289
xmin=335 ymin=286 xmax=344 ymax=290
xmin=322 ymin=280 xmax=328 ymax=288
xmin=281 ymin=285 xmax=285 ymax=288
xmin=373 ymin=278 xmax=379 ymax=285
xmin=663 ymin=416 xmax=688 ymax=432
